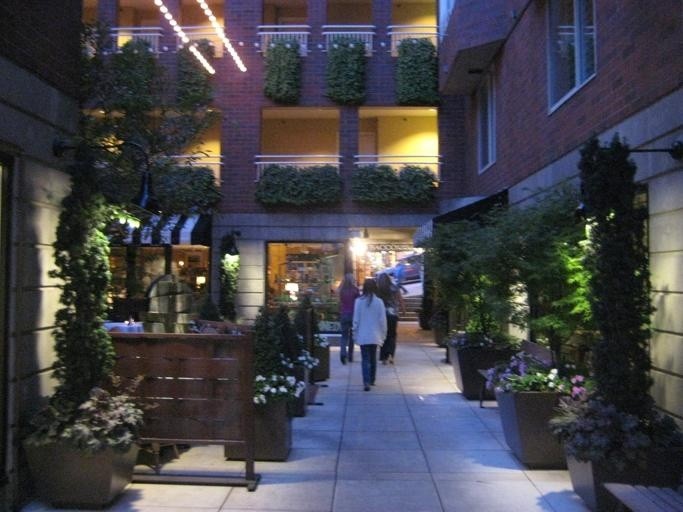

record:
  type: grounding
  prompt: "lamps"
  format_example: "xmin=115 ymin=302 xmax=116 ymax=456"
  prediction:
xmin=575 ymin=140 xmax=682 ymax=216
xmin=52 ymin=139 xmax=162 ymax=216
xmin=222 ymin=230 xmax=241 ymax=261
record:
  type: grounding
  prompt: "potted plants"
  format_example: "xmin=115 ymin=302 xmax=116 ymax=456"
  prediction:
xmin=19 ymin=171 xmax=158 ymax=511
xmin=549 ymin=131 xmax=682 ymax=512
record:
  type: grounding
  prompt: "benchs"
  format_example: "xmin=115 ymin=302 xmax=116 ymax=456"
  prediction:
xmin=603 ymin=480 xmax=683 ymax=511
xmin=476 ymin=341 xmax=560 ymax=409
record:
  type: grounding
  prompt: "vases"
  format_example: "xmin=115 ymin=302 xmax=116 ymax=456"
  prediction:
xmin=458 ymin=345 xmax=513 ymax=399
xmin=224 ymin=403 xmax=294 ymax=461
xmin=311 ymin=350 xmax=329 ymax=381
xmin=494 ymin=385 xmax=575 ymax=469
xmin=286 ymin=368 xmax=312 ymax=416
xmin=271 ymin=385 xmax=309 ymax=417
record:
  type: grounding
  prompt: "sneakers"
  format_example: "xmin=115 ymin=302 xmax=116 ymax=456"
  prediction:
xmin=364 ymin=382 xmax=369 ymax=391
xmin=382 ymin=360 xmax=394 ymax=366
xmin=340 ymin=355 xmax=352 ymax=365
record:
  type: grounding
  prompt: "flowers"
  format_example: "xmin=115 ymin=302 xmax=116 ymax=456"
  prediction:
xmin=433 ymin=194 xmax=521 ymax=347
xmin=240 ymin=306 xmax=305 ymax=405
xmin=271 ymin=302 xmax=321 ymax=370
xmin=295 ymin=294 xmax=330 ymax=349
xmin=485 ymin=181 xmax=586 ymax=403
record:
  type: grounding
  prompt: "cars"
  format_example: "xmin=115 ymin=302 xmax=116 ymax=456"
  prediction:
xmin=375 ymin=254 xmax=421 ymax=283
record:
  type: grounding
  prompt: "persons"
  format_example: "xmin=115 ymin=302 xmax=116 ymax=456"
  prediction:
xmin=336 ymin=273 xmax=360 ymax=363
xmin=390 ymin=261 xmax=407 ymax=294
xmin=389 ymin=273 xmax=399 ymax=287
xmin=376 ymin=272 xmax=406 ymax=364
xmin=352 ymin=280 xmax=387 ymax=390
